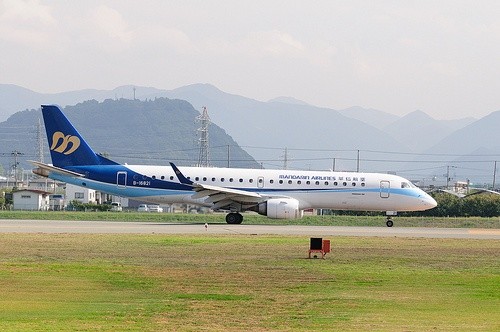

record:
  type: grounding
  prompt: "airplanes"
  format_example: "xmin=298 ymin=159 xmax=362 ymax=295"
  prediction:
xmin=26 ymin=105 xmax=438 ymax=227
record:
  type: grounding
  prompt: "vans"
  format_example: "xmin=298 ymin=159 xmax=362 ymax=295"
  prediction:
xmin=111 ymin=202 xmax=122 ymax=211
xmin=138 ymin=204 xmax=149 ymax=212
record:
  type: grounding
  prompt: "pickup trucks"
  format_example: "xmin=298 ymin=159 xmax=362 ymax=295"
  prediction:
xmin=150 ymin=205 xmax=163 ymax=212
